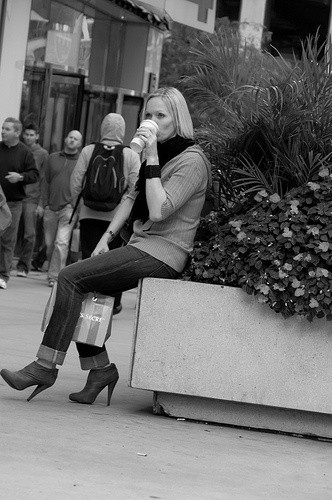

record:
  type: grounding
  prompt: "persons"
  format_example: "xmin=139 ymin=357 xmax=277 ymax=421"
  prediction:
xmin=71 ymin=114 xmax=142 ymax=314
xmin=0 ymin=86 xmax=212 ymax=406
xmin=15 ymin=124 xmax=49 ymax=277
xmin=43 ymin=130 xmax=82 ymax=287
xmin=0 ymin=116 xmax=40 ymax=289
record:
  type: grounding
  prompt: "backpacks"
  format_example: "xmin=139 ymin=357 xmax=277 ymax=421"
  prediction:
xmin=68 ymin=141 xmax=132 ymax=224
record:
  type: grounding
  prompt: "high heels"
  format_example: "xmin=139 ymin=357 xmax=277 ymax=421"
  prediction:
xmin=69 ymin=363 xmax=119 ymax=406
xmin=0 ymin=361 xmax=60 ymax=402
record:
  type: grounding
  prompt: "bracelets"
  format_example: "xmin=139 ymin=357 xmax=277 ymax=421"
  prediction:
xmin=106 ymin=230 xmax=115 ymax=242
xmin=144 ymin=165 xmax=161 ymax=179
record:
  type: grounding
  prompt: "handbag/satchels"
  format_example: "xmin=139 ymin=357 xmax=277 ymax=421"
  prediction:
xmin=41 ymin=272 xmax=116 ymax=347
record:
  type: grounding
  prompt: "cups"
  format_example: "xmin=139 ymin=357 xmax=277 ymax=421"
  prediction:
xmin=130 ymin=119 xmax=159 ymax=152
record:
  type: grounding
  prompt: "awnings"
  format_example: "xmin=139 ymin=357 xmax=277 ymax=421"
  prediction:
xmin=56 ymin=0 xmax=173 ymax=30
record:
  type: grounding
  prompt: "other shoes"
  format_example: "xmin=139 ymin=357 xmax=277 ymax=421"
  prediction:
xmin=17 ymin=271 xmax=27 ymax=278
xmin=32 ymin=261 xmax=38 ymax=271
xmin=0 ymin=279 xmax=6 ymax=289
xmin=48 ymin=281 xmax=54 ymax=287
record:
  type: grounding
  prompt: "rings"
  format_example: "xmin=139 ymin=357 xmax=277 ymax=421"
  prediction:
xmin=146 ymin=135 xmax=151 ymax=138
